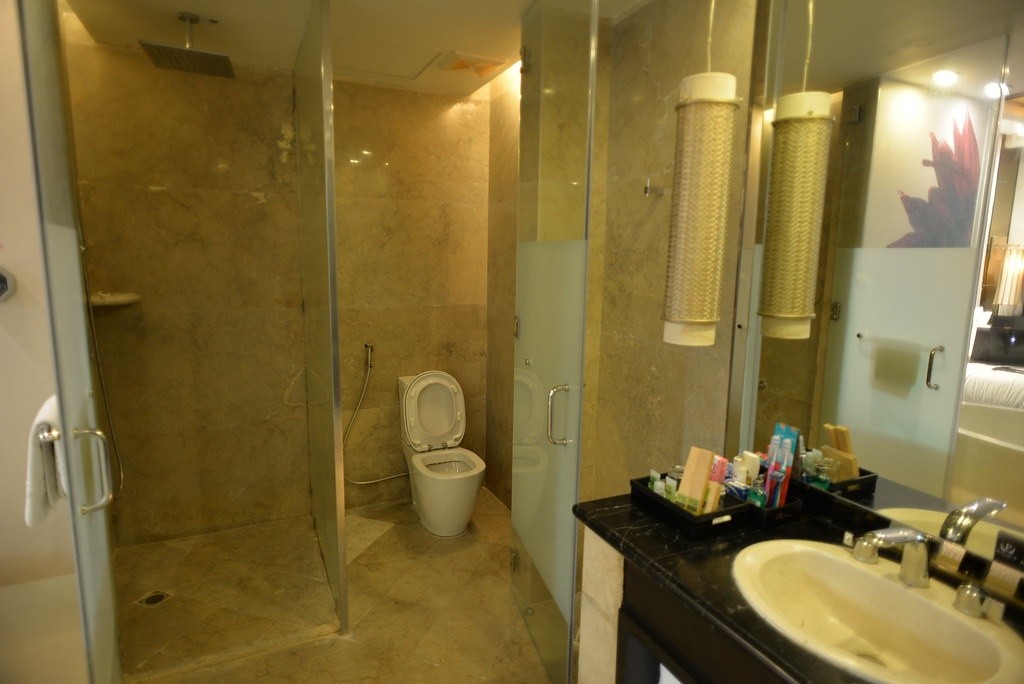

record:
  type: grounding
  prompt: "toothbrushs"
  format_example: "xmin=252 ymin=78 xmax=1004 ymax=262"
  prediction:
xmin=762 ymin=435 xmax=781 ymax=507
xmin=776 ymin=438 xmax=788 ymax=507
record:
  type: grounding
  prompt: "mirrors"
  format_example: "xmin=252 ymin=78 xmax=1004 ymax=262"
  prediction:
xmin=757 ymin=33 xmax=1009 ymax=247
xmin=941 ymin=91 xmax=1024 ymax=535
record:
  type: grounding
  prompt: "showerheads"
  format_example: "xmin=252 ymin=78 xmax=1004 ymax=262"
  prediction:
xmin=137 ymin=39 xmax=237 ymax=80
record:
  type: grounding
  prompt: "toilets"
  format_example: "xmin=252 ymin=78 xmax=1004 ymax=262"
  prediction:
xmin=512 ymin=365 xmax=548 ymax=531
xmin=399 ymin=369 xmax=487 ymax=538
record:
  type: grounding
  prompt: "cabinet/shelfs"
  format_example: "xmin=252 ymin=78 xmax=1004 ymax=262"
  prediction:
xmin=616 ymin=558 xmax=809 ymax=684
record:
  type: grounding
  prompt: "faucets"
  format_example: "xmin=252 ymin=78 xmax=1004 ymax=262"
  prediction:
xmin=939 ymin=496 xmax=1007 ymax=547
xmin=852 ymin=525 xmax=930 ymax=588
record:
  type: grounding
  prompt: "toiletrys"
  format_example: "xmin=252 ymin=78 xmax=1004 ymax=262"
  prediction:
xmin=798 ymin=435 xmax=841 ymax=492
xmin=730 ymin=455 xmax=768 ymax=508
xmin=648 ymin=469 xmax=677 ymax=504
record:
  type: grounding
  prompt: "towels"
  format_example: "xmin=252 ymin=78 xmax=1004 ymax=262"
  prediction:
xmin=24 ymin=394 xmax=71 ymax=529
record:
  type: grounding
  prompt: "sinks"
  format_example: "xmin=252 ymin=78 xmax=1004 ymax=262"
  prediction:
xmin=732 ymin=538 xmax=1024 ymax=684
xmin=874 ymin=508 xmax=1024 ymax=572
